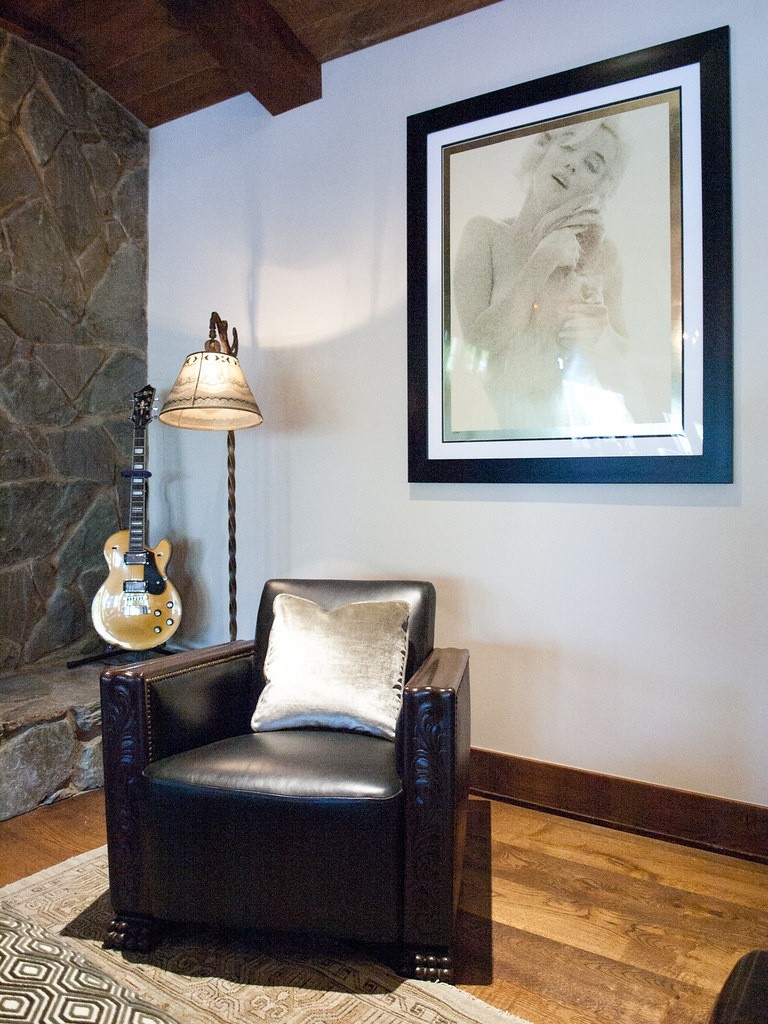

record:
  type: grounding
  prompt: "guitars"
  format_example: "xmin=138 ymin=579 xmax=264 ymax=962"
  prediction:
xmin=90 ymin=382 xmax=186 ymax=659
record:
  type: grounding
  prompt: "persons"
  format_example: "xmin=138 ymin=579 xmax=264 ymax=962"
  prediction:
xmin=452 ymin=116 xmax=635 ymax=433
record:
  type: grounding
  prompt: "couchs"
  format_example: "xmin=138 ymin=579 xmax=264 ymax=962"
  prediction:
xmin=99 ymin=578 xmax=471 ymax=986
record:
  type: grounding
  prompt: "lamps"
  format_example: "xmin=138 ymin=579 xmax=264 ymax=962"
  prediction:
xmin=158 ymin=311 xmax=264 ymax=644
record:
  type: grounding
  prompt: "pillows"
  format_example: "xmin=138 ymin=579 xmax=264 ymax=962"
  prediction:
xmin=250 ymin=593 xmax=410 ymax=743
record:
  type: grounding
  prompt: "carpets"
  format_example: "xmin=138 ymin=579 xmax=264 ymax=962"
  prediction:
xmin=0 ymin=842 xmax=531 ymax=1024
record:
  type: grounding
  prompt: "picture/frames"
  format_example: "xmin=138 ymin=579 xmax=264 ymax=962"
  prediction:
xmin=407 ymin=24 xmax=734 ymax=485
